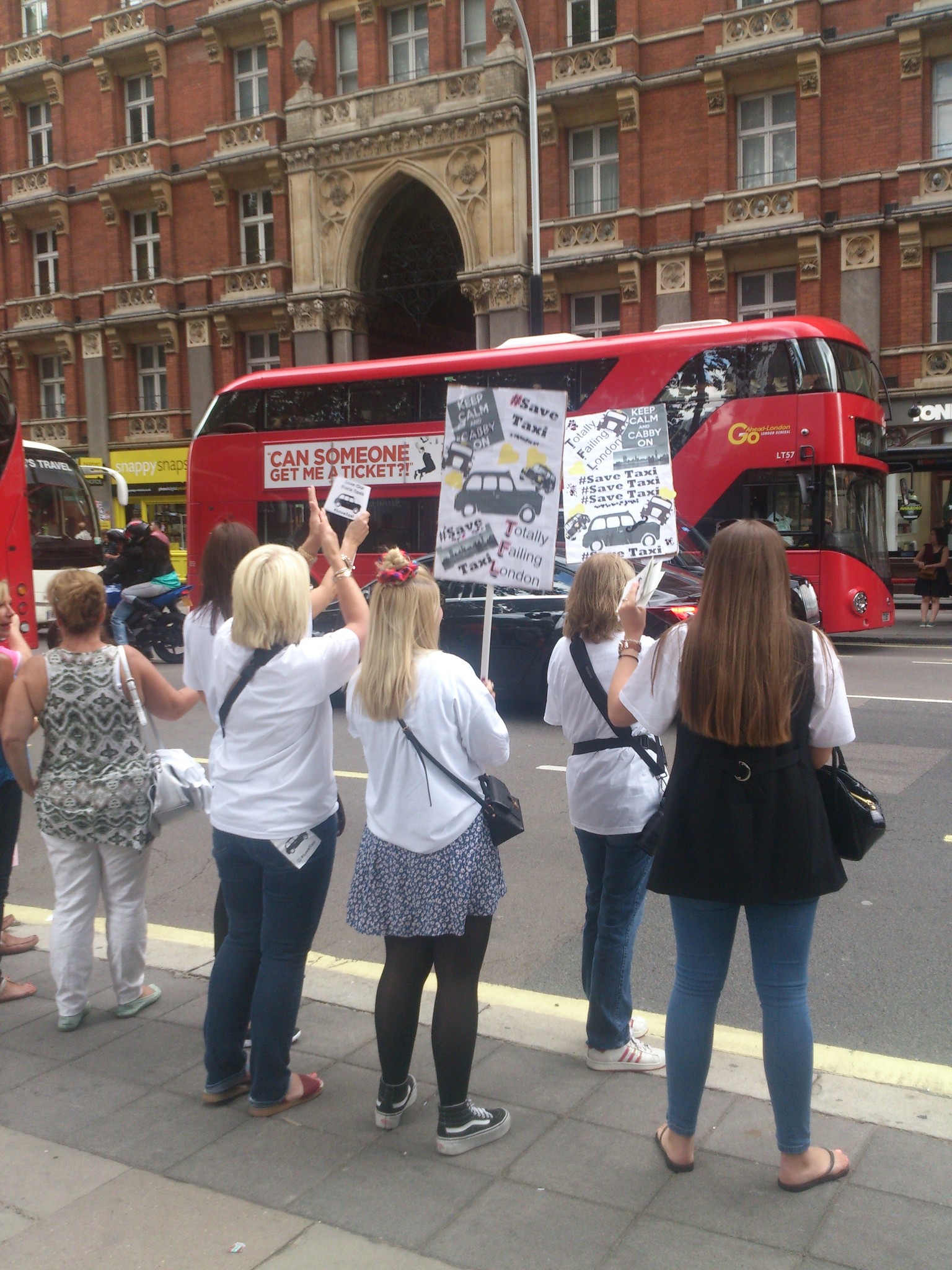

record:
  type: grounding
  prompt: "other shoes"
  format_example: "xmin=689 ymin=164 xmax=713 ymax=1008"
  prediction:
xmin=919 ymin=621 xmax=927 ymax=628
xmin=58 ymin=1000 xmax=91 ymax=1031
xmin=244 ymin=1026 xmax=301 ymax=1047
xmin=926 ymin=621 xmax=935 ymax=628
xmin=1 ymin=914 xmax=14 ymax=930
xmin=117 ymin=983 xmax=162 ymax=1018
xmin=0 ymin=932 xmax=40 ymax=956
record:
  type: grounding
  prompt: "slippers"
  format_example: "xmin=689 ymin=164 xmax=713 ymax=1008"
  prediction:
xmin=656 ymin=1124 xmax=694 ymax=1173
xmin=778 ymin=1147 xmax=849 ymax=1192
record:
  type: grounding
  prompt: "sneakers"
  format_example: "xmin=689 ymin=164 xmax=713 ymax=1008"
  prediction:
xmin=586 ymin=1034 xmax=666 ymax=1071
xmin=375 ymin=1071 xmax=418 ymax=1130
xmin=628 ymin=1015 xmax=648 ymax=1037
xmin=436 ymin=1093 xmax=511 ymax=1154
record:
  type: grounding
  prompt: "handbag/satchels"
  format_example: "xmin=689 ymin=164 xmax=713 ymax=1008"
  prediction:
xmin=918 ymin=567 xmax=938 ymax=580
xmin=816 ymin=744 xmax=885 ymax=860
xmin=336 ymin=798 xmax=346 ymax=838
xmin=116 ymin=645 xmax=207 ymax=824
xmin=479 ymin=775 xmax=525 ymax=848
xmin=634 ymin=808 xmax=666 ymax=857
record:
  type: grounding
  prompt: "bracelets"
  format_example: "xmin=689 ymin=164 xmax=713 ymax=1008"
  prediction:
xmin=340 ymin=553 xmax=355 ymax=569
xmin=298 ymin=545 xmax=318 ymax=564
xmin=332 ymin=568 xmax=352 ymax=582
xmin=25 ymin=778 xmax=33 ymax=794
xmin=617 ymin=639 xmax=643 ymax=662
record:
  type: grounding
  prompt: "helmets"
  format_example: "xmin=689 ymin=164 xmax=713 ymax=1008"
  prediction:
xmin=122 ymin=519 xmax=150 ymax=542
xmin=106 ymin=529 xmax=127 ymax=555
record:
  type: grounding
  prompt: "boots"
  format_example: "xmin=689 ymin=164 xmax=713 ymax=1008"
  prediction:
xmin=132 ymin=598 xmax=161 ymax=626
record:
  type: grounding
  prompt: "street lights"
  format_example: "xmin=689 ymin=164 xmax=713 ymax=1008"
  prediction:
xmin=167 ymin=512 xmax=184 ymax=551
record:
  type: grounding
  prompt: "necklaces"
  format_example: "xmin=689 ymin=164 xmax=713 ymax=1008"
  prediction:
xmin=933 ymin=547 xmax=938 ymax=550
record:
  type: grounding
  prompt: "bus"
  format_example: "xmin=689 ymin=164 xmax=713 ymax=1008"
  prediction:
xmin=0 ymin=373 xmax=40 ymax=655
xmin=21 ymin=439 xmax=130 ymax=625
xmin=185 ymin=314 xmax=914 ymax=702
xmin=149 ymin=510 xmax=187 ymax=537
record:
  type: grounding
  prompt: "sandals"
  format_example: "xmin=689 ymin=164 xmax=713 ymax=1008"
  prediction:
xmin=0 ymin=976 xmax=37 ymax=1001
xmin=202 ymin=1071 xmax=251 ymax=1102
xmin=250 ymin=1072 xmax=324 ymax=1117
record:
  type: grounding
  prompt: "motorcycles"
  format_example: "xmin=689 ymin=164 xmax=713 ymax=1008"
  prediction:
xmin=48 ymin=584 xmax=193 ymax=664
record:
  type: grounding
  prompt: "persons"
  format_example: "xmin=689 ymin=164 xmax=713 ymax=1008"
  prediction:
xmin=346 ymin=547 xmax=525 ymax=1157
xmin=73 ymin=521 xmax=93 ymax=540
xmin=0 ymin=580 xmax=39 ymax=1003
xmin=913 ymin=526 xmax=949 ymax=628
xmin=97 ymin=518 xmax=195 ymax=664
xmin=544 ymin=550 xmax=670 ymax=1072
xmin=1 ymin=570 xmax=213 ymax=1032
xmin=608 ymin=518 xmax=886 ymax=1193
xmin=725 ymin=494 xmax=832 ymax=547
xmin=183 ymin=485 xmax=370 ymax=1118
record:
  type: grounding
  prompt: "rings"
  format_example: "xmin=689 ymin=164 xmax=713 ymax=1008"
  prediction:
xmin=622 ymin=598 xmax=628 ymax=602
xmin=364 ymin=519 xmax=369 ymax=522
xmin=492 ymin=682 xmax=494 ymax=688
